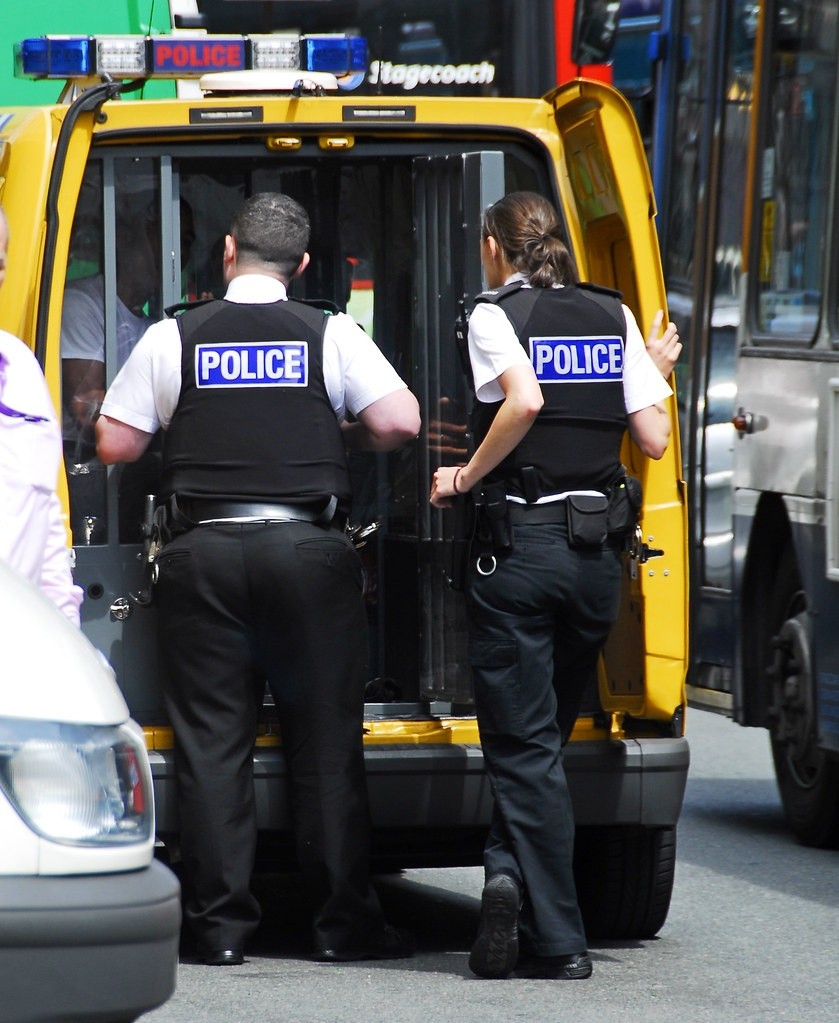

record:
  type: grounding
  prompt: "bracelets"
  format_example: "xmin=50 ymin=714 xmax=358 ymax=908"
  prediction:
xmin=454 ymin=468 xmax=461 ymax=495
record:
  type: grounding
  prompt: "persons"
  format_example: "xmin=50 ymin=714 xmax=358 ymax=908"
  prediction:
xmin=97 ymin=193 xmax=421 ymax=962
xmin=60 ymin=189 xmax=194 ymax=465
xmin=431 ymin=193 xmax=683 ymax=976
xmin=0 ymin=213 xmax=82 ymax=627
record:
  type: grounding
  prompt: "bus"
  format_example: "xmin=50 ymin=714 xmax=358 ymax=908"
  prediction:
xmin=572 ymin=0 xmax=839 ymax=851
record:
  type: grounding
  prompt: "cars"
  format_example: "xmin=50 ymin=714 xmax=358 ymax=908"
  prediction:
xmin=2 ymin=558 xmax=180 ymax=1022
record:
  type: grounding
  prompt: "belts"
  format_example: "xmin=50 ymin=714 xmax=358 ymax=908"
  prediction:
xmin=190 ymin=500 xmax=344 ymax=532
xmin=476 ymin=501 xmax=568 ymax=527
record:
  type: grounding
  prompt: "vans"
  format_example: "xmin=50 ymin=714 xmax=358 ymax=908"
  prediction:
xmin=1 ymin=33 xmax=695 ymax=945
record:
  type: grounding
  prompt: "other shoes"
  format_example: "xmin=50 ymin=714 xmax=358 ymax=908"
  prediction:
xmin=192 ymin=943 xmax=244 ymax=964
xmin=309 ymin=945 xmax=351 ymax=961
xmin=508 ymin=950 xmax=592 ymax=979
xmin=469 ymin=869 xmax=524 ymax=979
